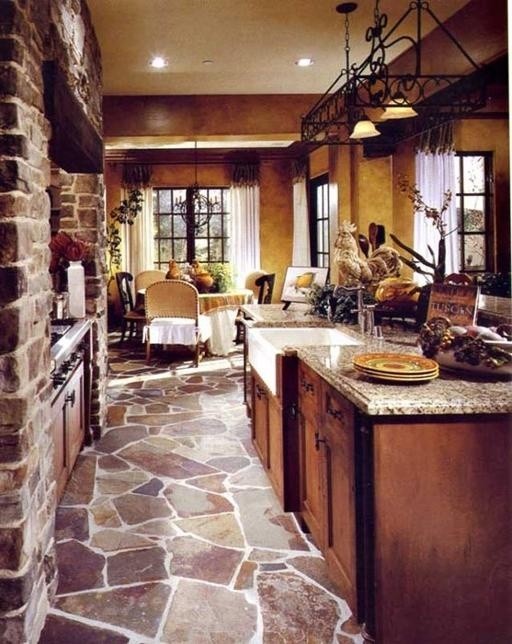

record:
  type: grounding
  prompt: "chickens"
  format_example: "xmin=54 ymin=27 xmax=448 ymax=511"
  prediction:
xmin=333 ymin=220 xmax=402 ymax=288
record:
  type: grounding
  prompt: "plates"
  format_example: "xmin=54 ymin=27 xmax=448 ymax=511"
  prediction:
xmin=350 ymin=352 xmax=441 ymax=384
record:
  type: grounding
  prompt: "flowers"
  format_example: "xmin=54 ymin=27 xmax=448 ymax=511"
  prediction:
xmin=49 ymin=230 xmax=90 ymax=272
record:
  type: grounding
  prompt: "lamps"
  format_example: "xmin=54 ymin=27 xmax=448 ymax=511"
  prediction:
xmin=169 ymin=139 xmax=222 ymax=233
xmin=301 ymin=1 xmax=492 ymax=149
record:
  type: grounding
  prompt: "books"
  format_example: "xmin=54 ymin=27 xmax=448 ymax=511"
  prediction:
xmin=425 ymin=282 xmax=480 ymax=327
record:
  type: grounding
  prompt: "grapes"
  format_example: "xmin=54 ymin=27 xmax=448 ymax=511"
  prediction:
xmin=421 ymin=319 xmax=487 ymax=366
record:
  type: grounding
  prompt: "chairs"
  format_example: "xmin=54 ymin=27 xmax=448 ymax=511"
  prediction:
xmin=114 ymin=270 xmax=275 ymax=368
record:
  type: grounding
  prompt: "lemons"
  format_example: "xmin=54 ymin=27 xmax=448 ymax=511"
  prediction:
xmin=296 ymin=273 xmax=313 ymax=288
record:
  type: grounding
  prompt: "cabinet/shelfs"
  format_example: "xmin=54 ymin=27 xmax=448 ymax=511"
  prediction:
xmin=294 ymin=356 xmax=512 ymax=644
xmin=51 ymin=359 xmax=88 ymax=507
xmin=250 ymin=364 xmax=298 ymax=513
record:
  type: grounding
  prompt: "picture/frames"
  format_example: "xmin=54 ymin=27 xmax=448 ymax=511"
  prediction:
xmin=279 ymin=265 xmax=330 ymax=303
